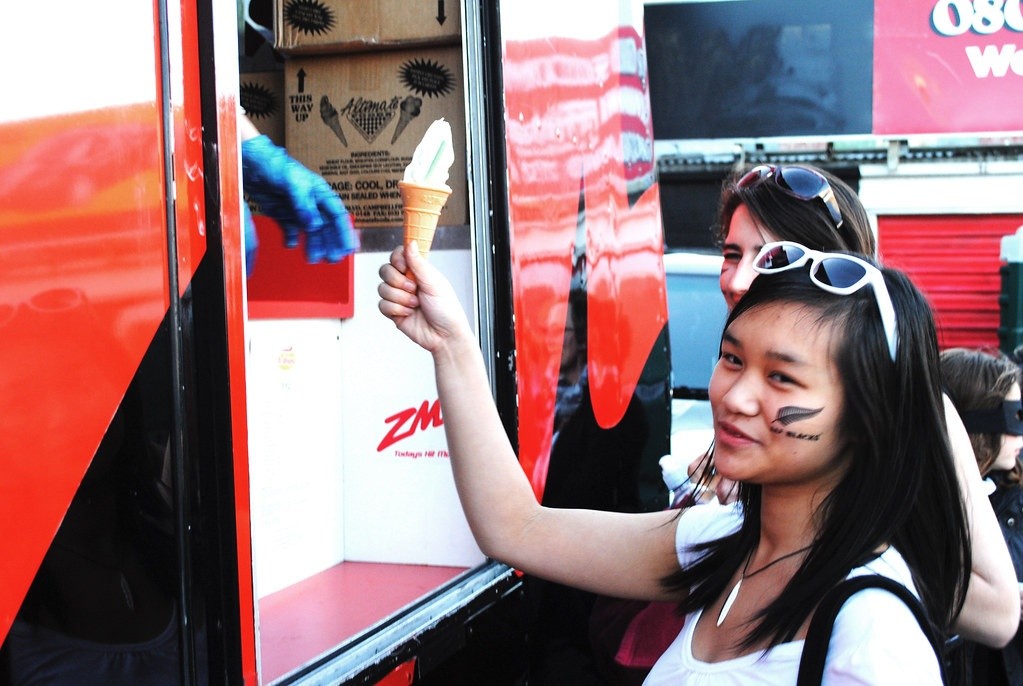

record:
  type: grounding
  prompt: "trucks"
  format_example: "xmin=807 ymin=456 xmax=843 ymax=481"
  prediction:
xmin=0 ymin=0 xmax=689 ymax=685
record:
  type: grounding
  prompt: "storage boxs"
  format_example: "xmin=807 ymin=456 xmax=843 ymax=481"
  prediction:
xmin=239 ymin=70 xmax=285 ymax=150
xmin=283 ymin=43 xmax=469 ymax=229
xmin=273 ymin=0 xmax=462 ymax=59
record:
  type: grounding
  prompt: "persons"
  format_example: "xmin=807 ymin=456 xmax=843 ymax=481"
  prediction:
xmin=960 ymin=345 xmax=1023 ymax=685
xmin=689 ymin=163 xmax=1023 ymax=652
xmin=930 ymin=345 xmax=1022 ymax=495
xmin=377 ymin=235 xmax=976 ymax=686
xmin=239 ymin=104 xmax=363 ymax=273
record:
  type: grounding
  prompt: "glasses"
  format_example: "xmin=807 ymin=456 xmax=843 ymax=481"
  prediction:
xmin=752 ymin=242 xmax=903 ymax=363
xmin=736 ymin=162 xmax=844 ymax=230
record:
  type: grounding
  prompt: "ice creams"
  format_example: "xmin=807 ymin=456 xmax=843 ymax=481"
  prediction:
xmin=395 ymin=118 xmax=455 ymax=302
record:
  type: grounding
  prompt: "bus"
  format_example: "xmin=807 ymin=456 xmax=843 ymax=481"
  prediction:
xmin=637 ymin=0 xmax=1022 ymax=459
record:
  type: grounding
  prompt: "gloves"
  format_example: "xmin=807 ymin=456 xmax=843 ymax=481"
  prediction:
xmin=240 ymin=133 xmax=361 ymax=274
